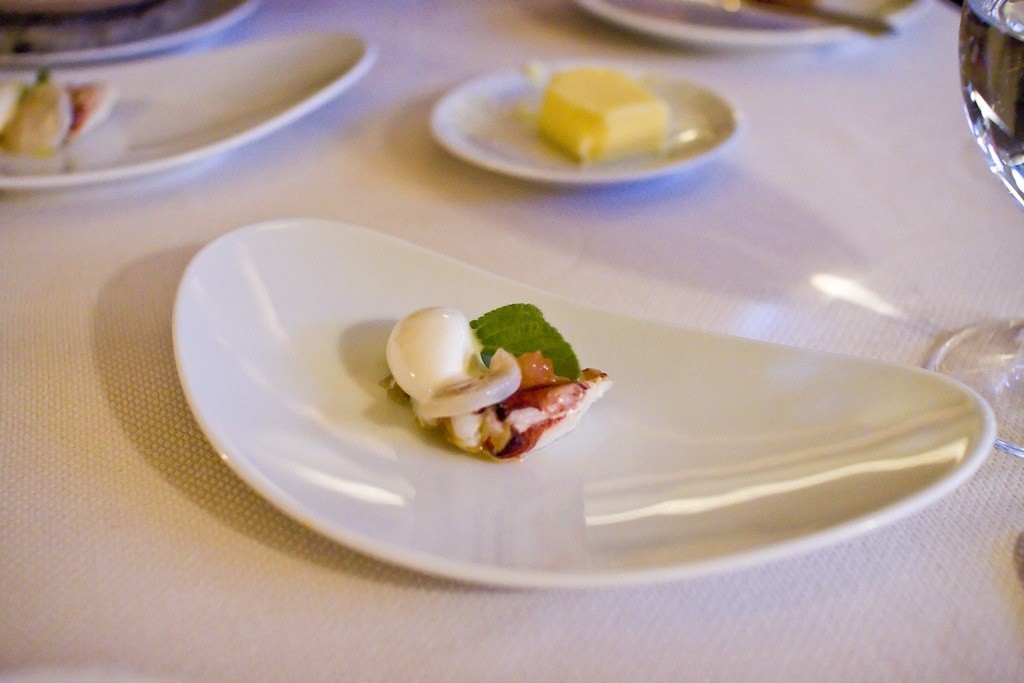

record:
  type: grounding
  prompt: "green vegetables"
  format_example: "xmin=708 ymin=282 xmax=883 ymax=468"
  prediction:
xmin=468 ymin=302 xmax=581 ymax=381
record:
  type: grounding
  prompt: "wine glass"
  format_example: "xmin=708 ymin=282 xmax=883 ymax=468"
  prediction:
xmin=928 ymin=0 xmax=1024 ymax=458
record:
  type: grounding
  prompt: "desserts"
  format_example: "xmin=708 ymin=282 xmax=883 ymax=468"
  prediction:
xmin=534 ymin=68 xmax=665 ymax=165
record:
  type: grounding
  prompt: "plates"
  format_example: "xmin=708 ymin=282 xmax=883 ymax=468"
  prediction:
xmin=576 ymin=0 xmax=935 ymax=49
xmin=169 ymin=218 xmax=995 ymax=592
xmin=0 ymin=0 xmax=267 ymax=66
xmin=0 ymin=31 xmax=380 ymax=191
xmin=427 ymin=60 xmax=746 ymax=188
